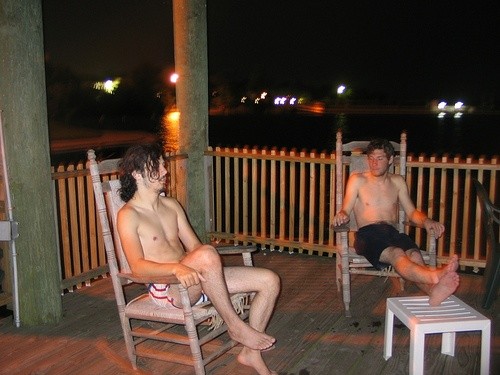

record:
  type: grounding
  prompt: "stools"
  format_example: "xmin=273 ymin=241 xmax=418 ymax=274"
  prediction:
xmin=384 ymin=294 xmax=491 ymax=375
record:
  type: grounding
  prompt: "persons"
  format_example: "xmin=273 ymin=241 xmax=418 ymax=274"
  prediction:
xmin=332 ymin=139 xmax=460 ymax=307
xmin=115 ymin=143 xmax=281 ymax=375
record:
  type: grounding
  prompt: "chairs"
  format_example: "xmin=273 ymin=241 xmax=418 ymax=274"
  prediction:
xmin=331 ymin=130 xmax=437 ymax=318
xmin=87 ymin=149 xmax=257 ymax=375
xmin=471 ymin=177 xmax=500 ymax=309
